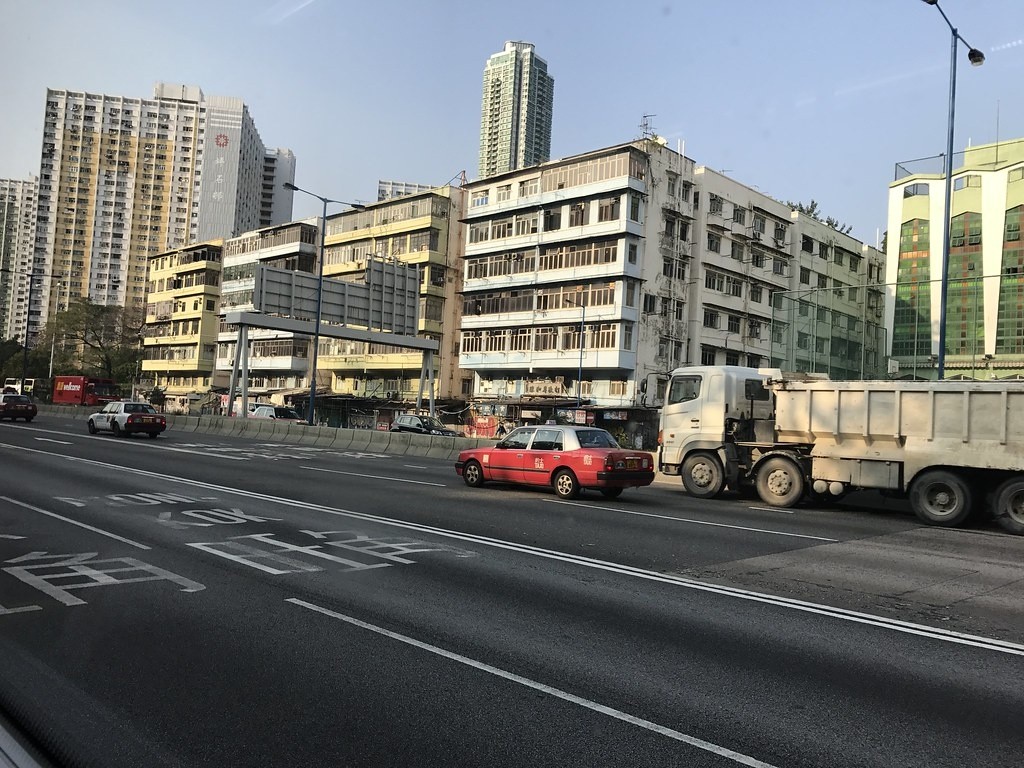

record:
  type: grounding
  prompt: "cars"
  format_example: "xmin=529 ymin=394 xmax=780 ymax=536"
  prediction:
xmin=455 ymin=420 xmax=655 ymax=500
xmin=0 ymin=394 xmax=37 ymax=422
xmin=247 ymin=406 xmax=308 ymax=423
xmin=87 ymin=399 xmax=166 ymax=438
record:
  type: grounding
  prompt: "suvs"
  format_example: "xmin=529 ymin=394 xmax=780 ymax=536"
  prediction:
xmin=390 ymin=415 xmax=465 ymax=437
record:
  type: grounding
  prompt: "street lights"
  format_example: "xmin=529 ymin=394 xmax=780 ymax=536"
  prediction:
xmin=283 ymin=183 xmax=365 ymax=426
xmin=922 ymin=0 xmax=985 ymax=379
xmin=35 ymin=283 xmax=62 ymax=378
xmin=564 ymin=299 xmax=585 ymax=408
xmin=0 ymin=269 xmax=62 ymax=395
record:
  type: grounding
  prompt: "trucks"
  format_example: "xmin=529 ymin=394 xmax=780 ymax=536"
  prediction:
xmin=53 ymin=376 xmax=125 ymax=406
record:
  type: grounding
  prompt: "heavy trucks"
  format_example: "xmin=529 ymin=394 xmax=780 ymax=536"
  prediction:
xmin=657 ymin=365 xmax=1024 ymax=536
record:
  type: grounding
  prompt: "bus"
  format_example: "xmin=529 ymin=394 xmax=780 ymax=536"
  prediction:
xmin=4 ymin=378 xmax=52 ymax=401
